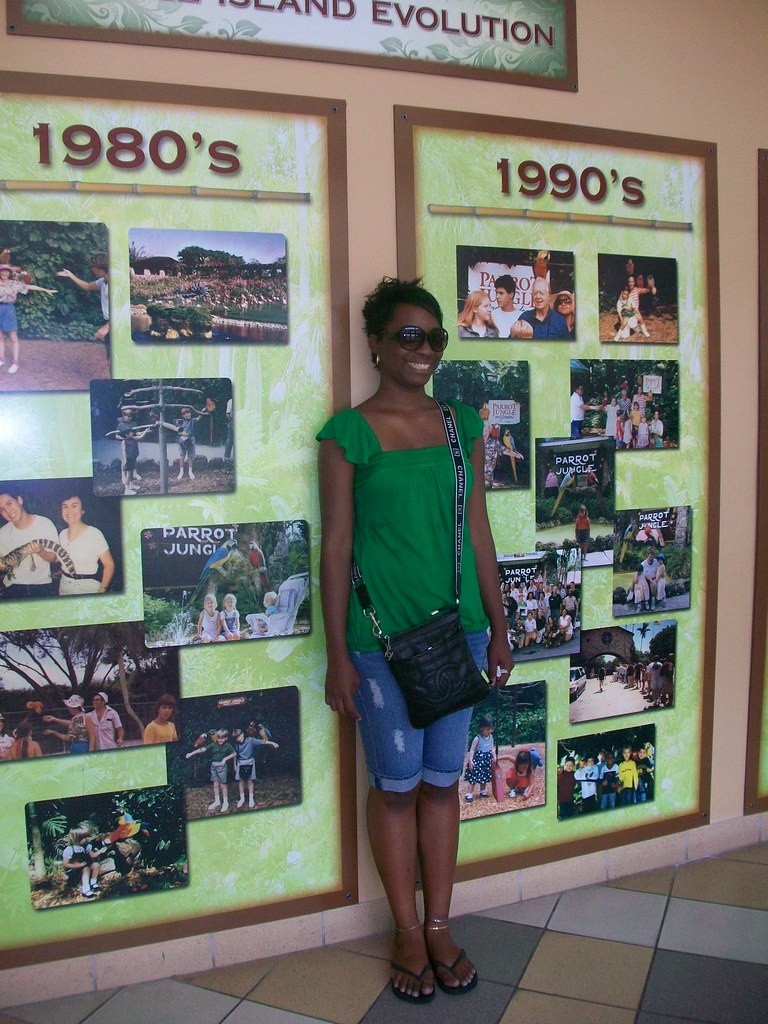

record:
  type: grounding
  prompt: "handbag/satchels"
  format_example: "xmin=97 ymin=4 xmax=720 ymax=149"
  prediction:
xmin=240 ymin=765 xmax=252 ymax=777
xmin=379 ymin=611 xmax=490 ymax=729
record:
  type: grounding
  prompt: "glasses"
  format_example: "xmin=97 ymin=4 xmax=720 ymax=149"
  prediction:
xmin=556 ymin=298 xmax=571 ymax=306
xmin=382 ymin=326 xmax=449 ymax=352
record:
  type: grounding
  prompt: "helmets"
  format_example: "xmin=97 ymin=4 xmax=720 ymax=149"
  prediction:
xmin=656 ymin=552 xmax=665 ymax=562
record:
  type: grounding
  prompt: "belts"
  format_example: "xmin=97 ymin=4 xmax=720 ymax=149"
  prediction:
xmin=62 ymin=570 xmax=96 ymax=579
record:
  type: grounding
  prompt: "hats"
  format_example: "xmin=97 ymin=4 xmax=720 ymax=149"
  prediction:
xmin=62 ymin=695 xmax=85 ymax=711
xmin=0 ymin=264 xmax=11 ymax=270
xmin=99 ymin=693 xmax=108 ymax=703
xmin=549 ymin=291 xmax=574 ymax=310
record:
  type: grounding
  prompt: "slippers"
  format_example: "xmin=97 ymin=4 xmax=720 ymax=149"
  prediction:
xmin=391 ymin=963 xmax=435 ymax=1005
xmin=432 ymin=949 xmax=479 ymax=995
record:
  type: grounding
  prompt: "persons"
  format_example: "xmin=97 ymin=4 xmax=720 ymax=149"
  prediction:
xmin=2 ymin=477 xmax=317 ymax=915
xmin=426 ymin=505 xmax=693 ymax=819
xmin=431 ymin=237 xmax=679 ymax=552
xmin=2 ymin=219 xmax=291 ymax=500
xmin=314 ymin=273 xmax=513 ymax=1002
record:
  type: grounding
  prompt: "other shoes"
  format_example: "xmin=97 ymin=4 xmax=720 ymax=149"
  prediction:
xmin=91 ymin=884 xmax=101 ymax=890
xmin=480 ymin=792 xmax=488 ymax=797
xmin=465 ymin=797 xmax=473 ymax=802
xmin=180 ymin=438 xmax=193 ymax=451
xmin=0 ymin=360 xmax=5 ymax=367
xmin=82 ymin=891 xmax=94 ymax=897
xmin=8 ymin=364 xmax=18 ymax=374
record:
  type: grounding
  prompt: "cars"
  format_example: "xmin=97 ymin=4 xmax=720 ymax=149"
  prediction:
xmin=570 ymin=667 xmax=587 ymax=699
